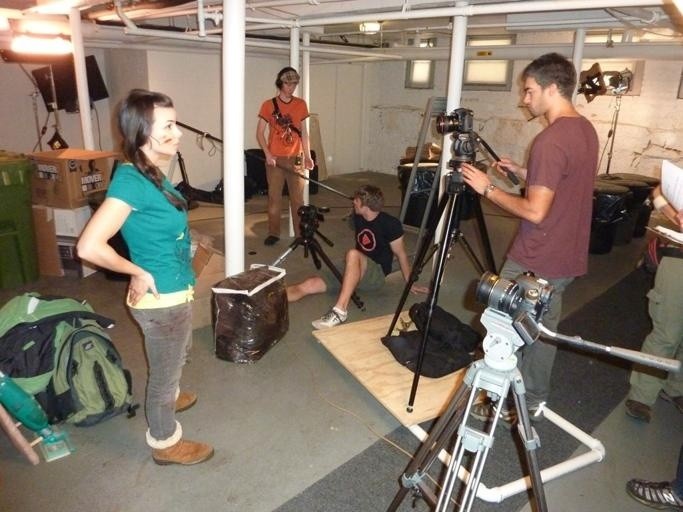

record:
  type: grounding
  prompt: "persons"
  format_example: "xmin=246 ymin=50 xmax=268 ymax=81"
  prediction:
xmin=622 ymin=161 xmax=683 ymax=420
xmin=283 ymin=184 xmax=431 ymax=329
xmin=460 ymin=53 xmax=599 ymax=431
xmin=625 ymin=444 xmax=682 ymax=511
xmin=74 ymin=87 xmax=215 ymax=466
xmin=254 ymin=66 xmax=314 ymax=247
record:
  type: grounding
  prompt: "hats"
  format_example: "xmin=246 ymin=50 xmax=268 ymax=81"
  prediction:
xmin=280 ymin=71 xmax=300 ymax=83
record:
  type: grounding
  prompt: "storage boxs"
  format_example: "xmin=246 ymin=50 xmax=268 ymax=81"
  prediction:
xmin=32 ymin=205 xmax=100 ymax=276
xmin=27 ymin=149 xmax=120 ymax=210
xmin=191 ymin=242 xmax=224 ymax=279
xmin=190 ymin=272 xmax=226 ymax=331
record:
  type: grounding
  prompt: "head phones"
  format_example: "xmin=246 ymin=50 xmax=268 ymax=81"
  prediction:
xmin=275 ymin=67 xmax=296 ymax=87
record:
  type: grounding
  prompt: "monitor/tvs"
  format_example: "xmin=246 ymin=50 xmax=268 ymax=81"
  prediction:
xmin=33 ymin=55 xmax=109 ymax=113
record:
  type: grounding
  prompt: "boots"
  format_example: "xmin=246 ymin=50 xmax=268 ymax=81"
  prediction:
xmin=175 ymin=386 xmax=197 ymax=412
xmin=146 ymin=420 xmax=214 ymax=465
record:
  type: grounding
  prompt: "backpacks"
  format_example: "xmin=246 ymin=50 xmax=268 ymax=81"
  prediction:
xmin=47 ymin=319 xmax=140 ymax=426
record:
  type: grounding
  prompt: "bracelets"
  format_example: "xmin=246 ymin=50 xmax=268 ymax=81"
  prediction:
xmin=652 ymin=194 xmax=669 ymax=213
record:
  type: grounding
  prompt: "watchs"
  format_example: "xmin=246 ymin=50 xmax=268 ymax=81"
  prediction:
xmin=482 ymin=183 xmax=498 ymax=199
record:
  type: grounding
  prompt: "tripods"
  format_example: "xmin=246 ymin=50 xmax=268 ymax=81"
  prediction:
xmin=272 ymin=236 xmax=367 ymax=315
xmin=385 ymin=371 xmax=548 ymax=512
xmin=387 ymin=194 xmax=497 ymax=412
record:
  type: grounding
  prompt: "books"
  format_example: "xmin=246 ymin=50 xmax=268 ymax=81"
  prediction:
xmin=32 ymin=424 xmax=69 ymax=462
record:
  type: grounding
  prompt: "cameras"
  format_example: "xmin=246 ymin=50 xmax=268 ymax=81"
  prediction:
xmin=298 ymin=205 xmax=329 ymax=223
xmin=473 ymin=270 xmax=554 ymax=345
xmin=437 ymin=105 xmax=476 ymax=134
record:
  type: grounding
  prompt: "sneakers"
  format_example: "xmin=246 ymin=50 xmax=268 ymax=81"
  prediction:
xmin=470 ymin=401 xmax=543 ymax=429
xmin=627 ymin=479 xmax=683 ymax=512
xmin=312 ymin=304 xmax=347 ymax=329
xmin=264 ymin=235 xmax=279 ymax=245
xmin=625 ymin=389 xmax=683 ymax=424
